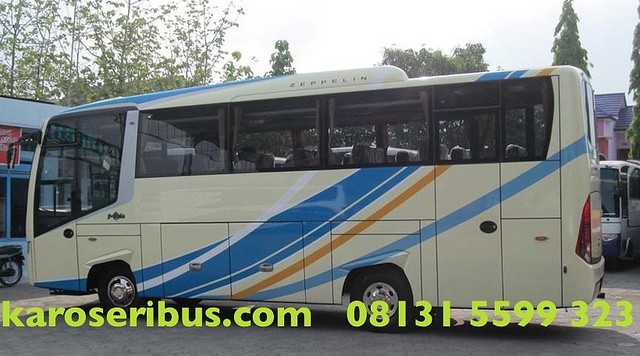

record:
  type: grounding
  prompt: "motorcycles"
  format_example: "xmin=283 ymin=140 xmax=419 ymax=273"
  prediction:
xmin=0 ymin=245 xmax=25 ymax=287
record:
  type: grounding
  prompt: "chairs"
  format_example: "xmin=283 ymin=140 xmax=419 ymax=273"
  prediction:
xmin=352 ymin=145 xmax=384 ymax=164
xmin=439 ymin=143 xmax=448 ymax=160
xmin=387 ymin=151 xmax=419 ymax=163
xmin=505 ymin=144 xmax=527 ymax=159
xmin=450 ymin=146 xmax=471 ymax=160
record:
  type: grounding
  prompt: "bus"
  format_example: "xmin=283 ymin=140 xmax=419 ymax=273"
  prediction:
xmin=25 ymin=64 xmax=605 ymax=333
xmin=599 ymin=158 xmax=640 ymax=272
